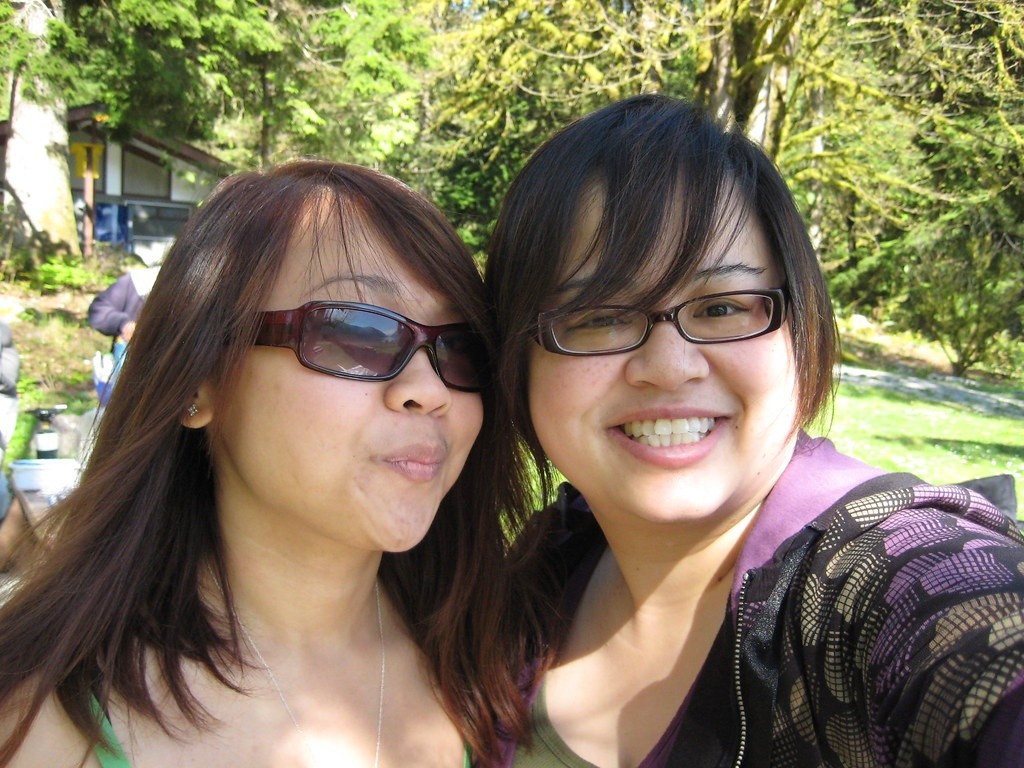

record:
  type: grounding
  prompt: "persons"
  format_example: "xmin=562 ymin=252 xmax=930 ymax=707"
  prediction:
xmin=475 ymin=93 xmax=1024 ymax=768
xmin=86 ymin=263 xmax=161 ymax=340
xmin=0 ymin=158 xmax=532 ymax=767
xmin=0 ymin=325 xmax=19 ymax=472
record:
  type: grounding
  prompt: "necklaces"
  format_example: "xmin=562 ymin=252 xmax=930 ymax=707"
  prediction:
xmin=203 ymin=560 xmax=386 ymax=766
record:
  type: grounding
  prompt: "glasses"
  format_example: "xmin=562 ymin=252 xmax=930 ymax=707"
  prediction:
xmin=223 ymin=299 xmax=483 ymax=392
xmin=527 ymin=280 xmax=792 ymax=354
